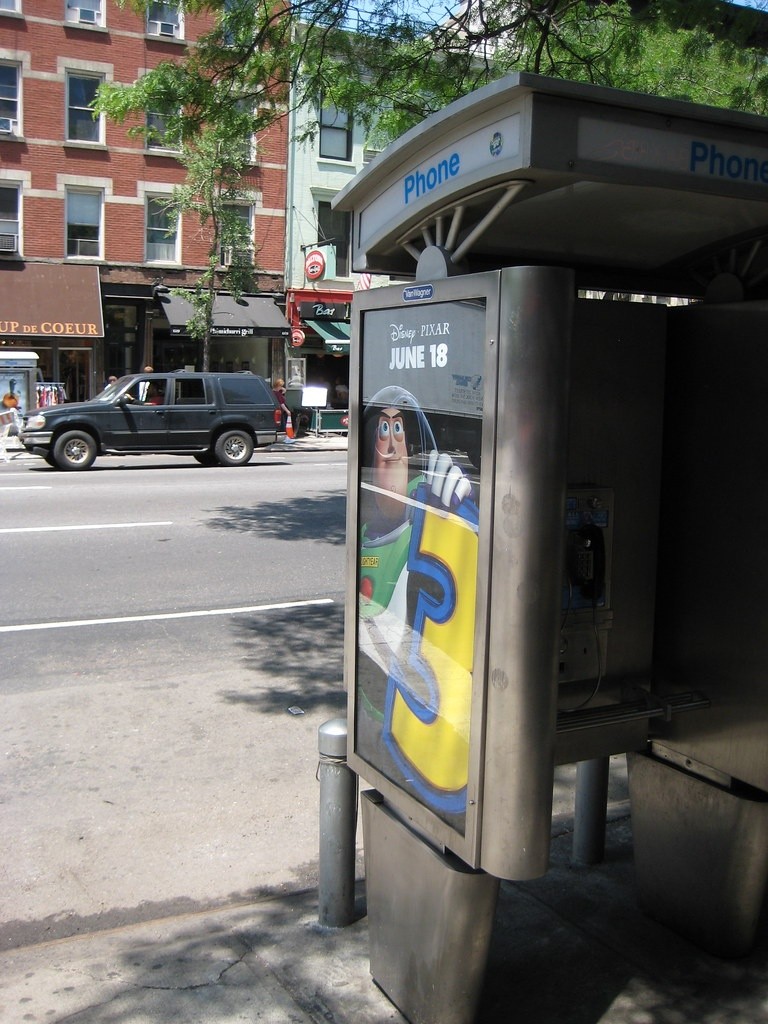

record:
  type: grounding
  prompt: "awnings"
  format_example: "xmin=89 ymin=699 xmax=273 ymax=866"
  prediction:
xmin=0 ymin=261 xmax=105 ymax=338
xmin=157 ymin=292 xmax=292 ymax=338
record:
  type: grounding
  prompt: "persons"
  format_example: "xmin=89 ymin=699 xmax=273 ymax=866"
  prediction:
xmin=272 ymin=379 xmax=300 ymax=437
xmin=124 ymin=381 xmax=163 ymax=406
xmin=100 ymin=376 xmax=118 ymax=400
xmin=304 ymin=320 xmax=350 ymax=352
xmin=139 ymin=366 xmax=154 ymax=401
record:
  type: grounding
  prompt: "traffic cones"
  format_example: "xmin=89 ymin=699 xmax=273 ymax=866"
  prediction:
xmin=284 ymin=412 xmax=295 ymax=439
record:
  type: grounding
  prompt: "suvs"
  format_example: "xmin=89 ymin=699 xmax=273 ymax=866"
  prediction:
xmin=18 ymin=369 xmax=283 ymax=471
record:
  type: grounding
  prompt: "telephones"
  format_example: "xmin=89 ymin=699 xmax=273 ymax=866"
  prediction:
xmin=560 ymin=483 xmax=614 ymax=634
